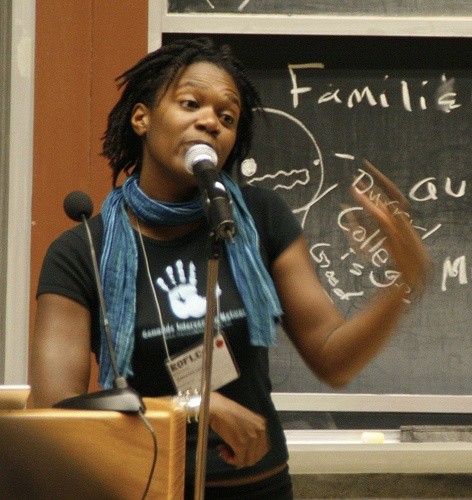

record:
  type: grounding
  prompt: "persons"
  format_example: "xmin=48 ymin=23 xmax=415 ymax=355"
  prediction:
xmin=27 ymin=39 xmax=434 ymax=500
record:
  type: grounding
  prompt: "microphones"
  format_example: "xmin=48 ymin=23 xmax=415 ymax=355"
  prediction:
xmin=184 ymin=144 xmax=237 ymax=244
xmin=51 ymin=189 xmax=146 ymax=413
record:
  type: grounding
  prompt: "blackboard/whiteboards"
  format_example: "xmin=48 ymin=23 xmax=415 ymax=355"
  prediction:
xmin=161 ymin=31 xmax=471 ymax=396
xmin=169 ymin=1 xmax=472 ymax=17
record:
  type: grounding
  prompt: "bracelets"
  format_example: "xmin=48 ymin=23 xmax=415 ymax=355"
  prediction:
xmin=174 ymin=386 xmax=201 ymax=424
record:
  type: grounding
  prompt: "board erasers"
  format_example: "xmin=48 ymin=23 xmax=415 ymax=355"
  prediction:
xmin=399 ymin=425 xmax=472 ymax=443
